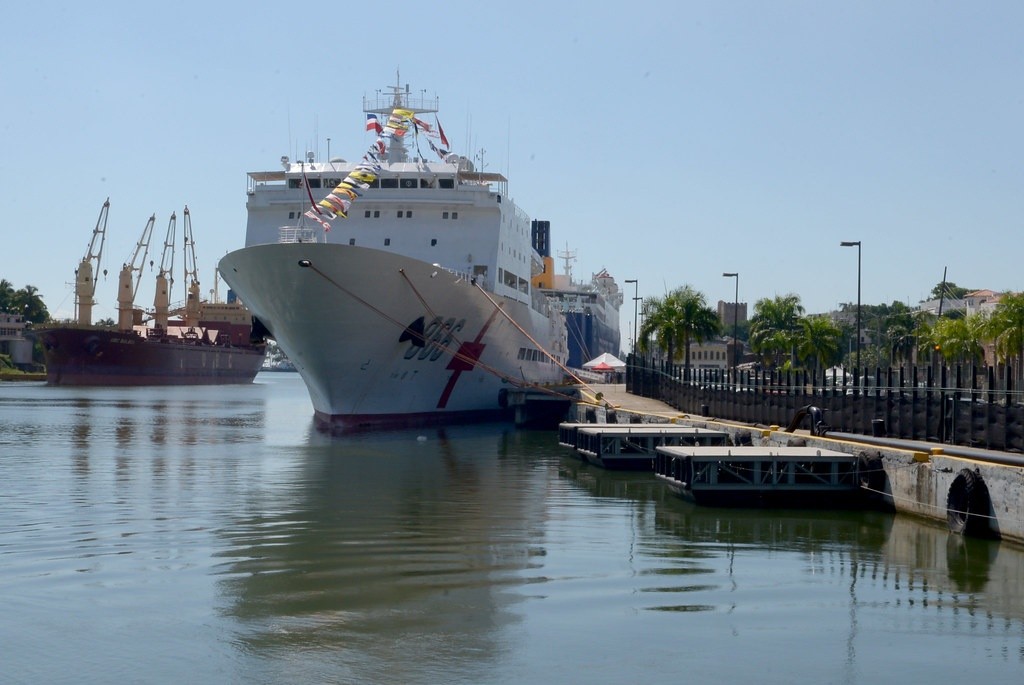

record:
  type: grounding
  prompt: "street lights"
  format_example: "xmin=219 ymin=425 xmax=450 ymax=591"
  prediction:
xmin=839 ymin=240 xmax=861 ymax=376
xmin=632 ymin=297 xmax=644 ymax=326
xmin=624 ymin=279 xmax=638 ymax=394
xmin=723 ymin=273 xmax=738 ymax=393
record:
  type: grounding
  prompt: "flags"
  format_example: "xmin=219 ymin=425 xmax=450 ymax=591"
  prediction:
xmin=304 ymin=111 xmax=451 ymax=234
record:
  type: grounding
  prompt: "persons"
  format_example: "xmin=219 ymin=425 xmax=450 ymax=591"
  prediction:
xmin=583 ymin=368 xmax=623 ymax=385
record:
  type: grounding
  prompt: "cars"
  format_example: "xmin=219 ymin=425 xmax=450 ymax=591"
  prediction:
xmin=816 ymin=385 xmax=911 ymax=398
xmin=689 ymin=369 xmax=772 ymax=393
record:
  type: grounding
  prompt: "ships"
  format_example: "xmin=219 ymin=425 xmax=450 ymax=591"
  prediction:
xmin=216 ymin=67 xmax=569 ymax=427
xmin=535 ymin=269 xmax=623 ymax=387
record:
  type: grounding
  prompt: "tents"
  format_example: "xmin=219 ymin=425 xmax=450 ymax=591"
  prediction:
xmin=825 ymin=365 xmax=853 ymax=381
xmin=582 ymin=352 xmax=627 ymax=385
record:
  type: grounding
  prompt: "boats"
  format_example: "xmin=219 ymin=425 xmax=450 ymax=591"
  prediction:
xmin=530 ymin=246 xmax=543 ymax=279
xmin=25 ymin=200 xmax=268 ymax=388
